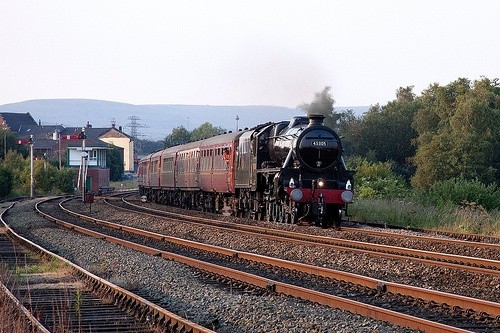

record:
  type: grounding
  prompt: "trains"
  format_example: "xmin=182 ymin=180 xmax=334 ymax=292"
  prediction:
xmin=137 ymin=114 xmax=357 ymax=229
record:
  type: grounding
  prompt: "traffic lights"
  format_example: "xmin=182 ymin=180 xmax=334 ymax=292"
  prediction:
xmin=77 ymin=133 xmax=86 ymax=140
xmin=27 ymin=138 xmax=35 ymax=145
